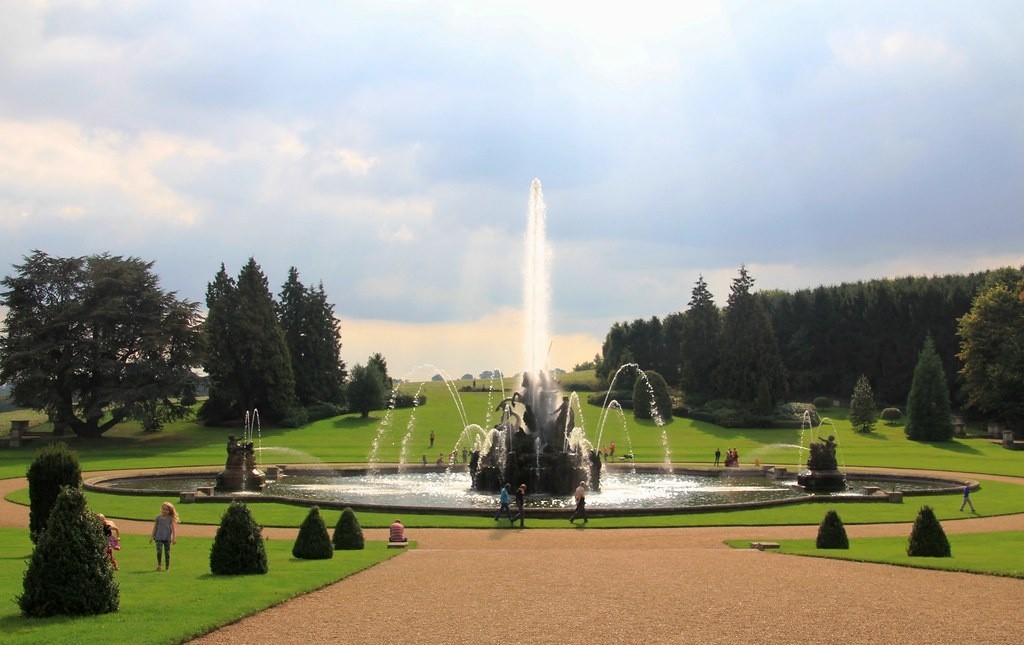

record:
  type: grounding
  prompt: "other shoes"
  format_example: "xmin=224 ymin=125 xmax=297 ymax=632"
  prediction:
xmin=569 ymin=519 xmax=573 ymax=524
xmin=157 ymin=566 xmax=162 ymax=571
xmin=510 ymin=518 xmax=513 ymax=526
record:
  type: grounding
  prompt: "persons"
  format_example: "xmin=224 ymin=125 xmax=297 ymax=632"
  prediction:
xmin=423 ymin=455 xmax=426 ymax=463
xmin=495 ymin=483 xmax=512 ymax=521
xmin=960 ymin=481 xmax=975 ymax=511
xmin=437 ymin=446 xmax=468 ymax=464
xmin=510 ymin=483 xmax=527 ymax=527
xmin=149 ymin=501 xmax=180 ymax=572
xmin=94 ymin=512 xmax=122 ymax=571
xmin=603 ymin=445 xmax=607 ymax=461
xmin=818 ymin=435 xmax=834 ymax=448
xmin=389 ymin=520 xmax=408 ymax=542
xmin=610 ymin=441 xmax=615 ymax=456
xmin=714 ymin=448 xmax=720 ymax=466
xmin=725 ymin=447 xmax=739 ymax=467
xmin=552 ymin=396 xmax=571 ymax=432
xmin=227 ymin=434 xmax=244 ymax=451
xmin=568 ymin=481 xmax=589 ymax=523
xmin=430 ymin=430 xmax=434 ymax=445
